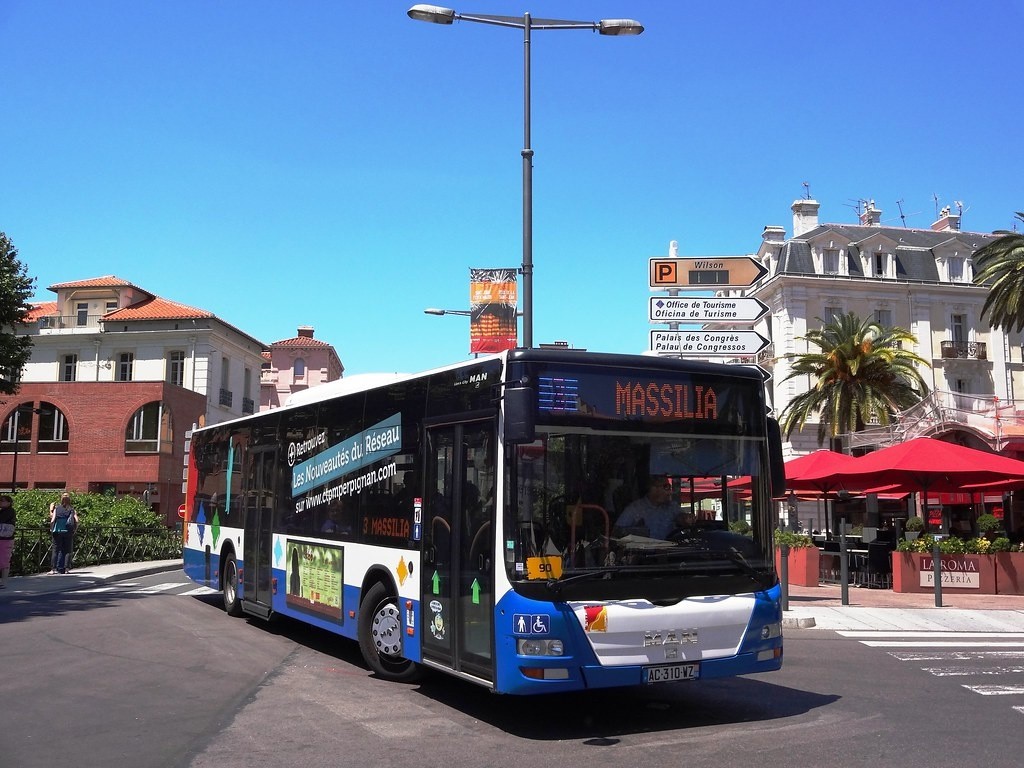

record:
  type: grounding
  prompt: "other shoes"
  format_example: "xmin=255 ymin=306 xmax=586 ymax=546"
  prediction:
xmin=46 ymin=568 xmax=57 ymax=576
xmin=0 ymin=582 xmax=7 ymax=590
xmin=61 ymin=568 xmax=70 ymax=574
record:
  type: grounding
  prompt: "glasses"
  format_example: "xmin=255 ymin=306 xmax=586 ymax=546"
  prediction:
xmin=650 ymin=482 xmax=671 ymax=491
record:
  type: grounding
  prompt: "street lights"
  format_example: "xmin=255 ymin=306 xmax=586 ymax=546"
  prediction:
xmin=425 ymin=308 xmax=523 ymax=362
xmin=407 ymin=1 xmax=644 ymax=347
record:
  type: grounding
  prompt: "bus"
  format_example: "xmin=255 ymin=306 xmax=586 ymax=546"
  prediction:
xmin=182 ymin=349 xmax=789 ymax=701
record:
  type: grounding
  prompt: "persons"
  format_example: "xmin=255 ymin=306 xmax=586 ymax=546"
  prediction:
xmin=610 ymin=473 xmax=696 ymax=540
xmin=386 ymin=472 xmax=419 ymax=522
xmin=0 ymin=494 xmax=17 ymax=589
xmin=319 ymin=499 xmax=353 ymax=536
xmin=46 ymin=493 xmax=79 ymax=576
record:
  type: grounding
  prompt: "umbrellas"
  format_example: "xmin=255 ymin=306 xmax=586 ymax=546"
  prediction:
xmin=667 ymin=437 xmax=1024 ymax=541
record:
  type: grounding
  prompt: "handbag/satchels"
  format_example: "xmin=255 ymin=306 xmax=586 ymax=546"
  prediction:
xmin=65 ymin=510 xmax=76 ymax=534
xmin=0 ymin=522 xmax=16 ymax=538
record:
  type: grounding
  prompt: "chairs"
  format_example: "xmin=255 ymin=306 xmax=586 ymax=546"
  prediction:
xmin=797 ymin=528 xmax=896 ymax=589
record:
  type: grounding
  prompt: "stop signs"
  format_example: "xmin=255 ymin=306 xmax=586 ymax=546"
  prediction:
xmin=178 ymin=504 xmax=186 ymax=518
